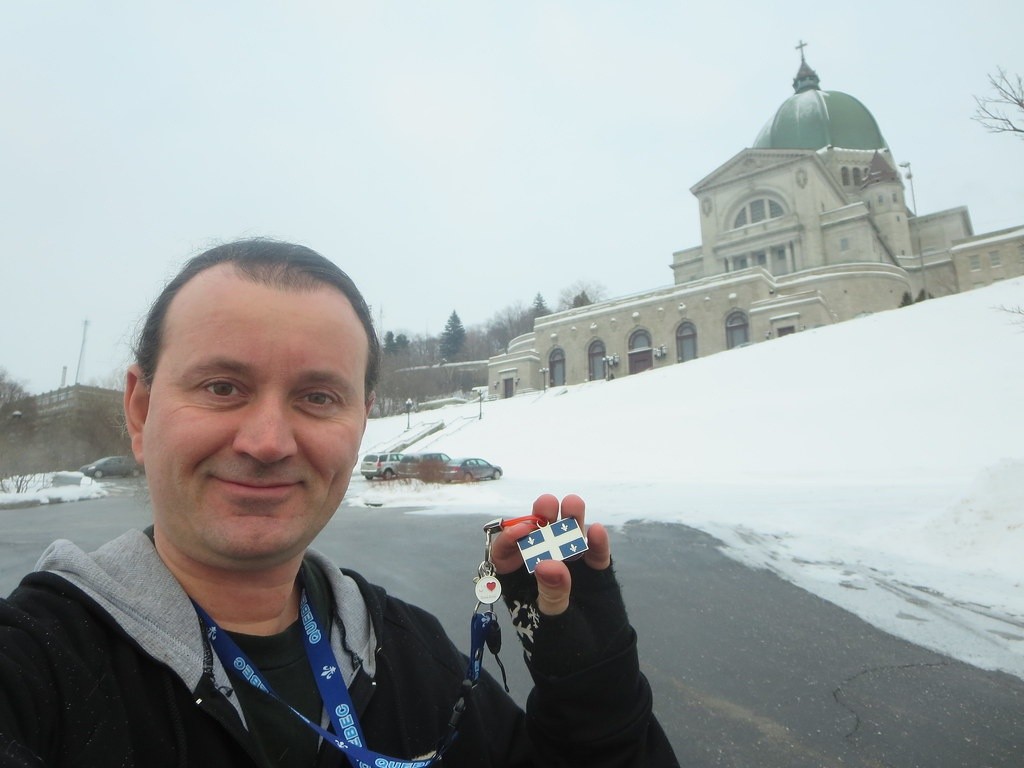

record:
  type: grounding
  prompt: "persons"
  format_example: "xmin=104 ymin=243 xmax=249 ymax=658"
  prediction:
xmin=0 ymin=241 xmax=683 ymax=768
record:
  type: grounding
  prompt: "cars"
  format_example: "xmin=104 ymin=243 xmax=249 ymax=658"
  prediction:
xmin=442 ymin=458 xmax=501 ymax=482
xmin=79 ymin=454 xmax=140 ymax=476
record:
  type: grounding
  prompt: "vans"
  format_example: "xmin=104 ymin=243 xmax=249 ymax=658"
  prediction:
xmin=398 ymin=453 xmax=451 ymax=481
xmin=361 ymin=453 xmax=408 ymax=481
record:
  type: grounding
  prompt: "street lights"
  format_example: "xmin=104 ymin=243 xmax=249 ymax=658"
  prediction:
xmin=899 ymin=158 xmax=929 ymax=300
xmin=477 ymin=388 xmax=485 ymax=419
xmin=406 ymin=398 xmax=414 ymax=431
xmin=601 ymin=354 xmax=613 ymax=379
xmin=538 ymin=366 xmax=550 ymax=391
